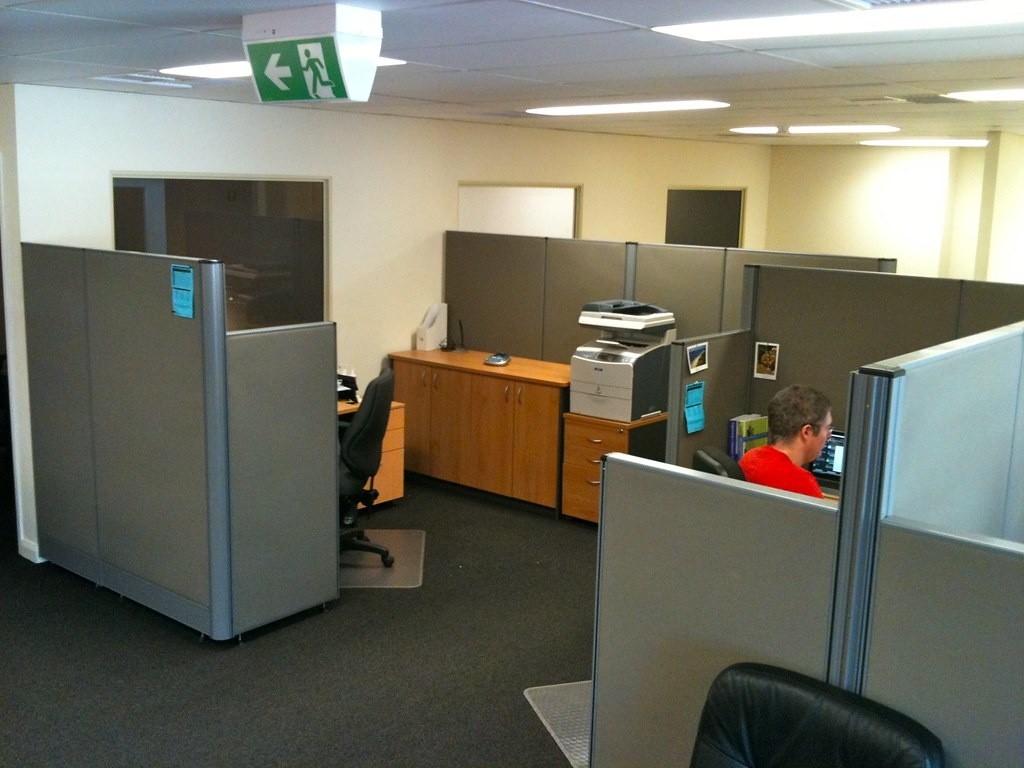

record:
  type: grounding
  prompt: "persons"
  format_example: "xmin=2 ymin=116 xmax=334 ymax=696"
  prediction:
xmin=735 ymin=384 xmax=833 ymax=500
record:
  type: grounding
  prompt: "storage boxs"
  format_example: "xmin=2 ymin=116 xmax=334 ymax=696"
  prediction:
xmin=415 ymin=302 xmax=449 ymax=351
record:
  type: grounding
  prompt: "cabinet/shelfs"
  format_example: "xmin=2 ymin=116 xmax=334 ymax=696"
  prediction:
xmin=559 ymin=412 xmax=669 ymax=524
xmin=337 ymin=394 xmax=405 ymax=515
xmin=390 ymin=347 xmax=572 ymax=517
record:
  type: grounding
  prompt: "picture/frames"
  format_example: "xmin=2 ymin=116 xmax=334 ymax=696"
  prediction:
xmin=688 ymin=341 xmax=709 ymax=374
xmin=753 ymin=342 xmax=780 ymax=381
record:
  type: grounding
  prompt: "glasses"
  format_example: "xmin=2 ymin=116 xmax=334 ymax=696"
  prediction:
xmin=793 ymin=423 xmax=834 ymax=436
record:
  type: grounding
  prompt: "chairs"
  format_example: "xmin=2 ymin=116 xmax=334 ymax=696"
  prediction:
xmin=694 ymin=662 xmax=946 ymax=768
xmin=337 ymin=369 xmax=397 ymax=571
xmin=697 ymin=445 xmax=750 ymax=482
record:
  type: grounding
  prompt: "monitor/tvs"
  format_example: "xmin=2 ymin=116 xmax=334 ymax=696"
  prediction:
xmin=809 ymin=430 xmax=846 ymax=483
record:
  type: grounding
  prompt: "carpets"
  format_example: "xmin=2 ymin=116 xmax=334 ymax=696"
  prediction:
xmin=335 ymin=528 xmax=427 ymax=590
xmin=526 ymin=680 xmax=593 ymax=768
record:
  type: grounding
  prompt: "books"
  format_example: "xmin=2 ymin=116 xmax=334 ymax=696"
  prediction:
xmin=730 ymin=414 xmax=769 ymax=459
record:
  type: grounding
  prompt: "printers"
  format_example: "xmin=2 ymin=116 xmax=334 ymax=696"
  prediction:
xmin=569 ymin=299 xmax=677 ymax=423
